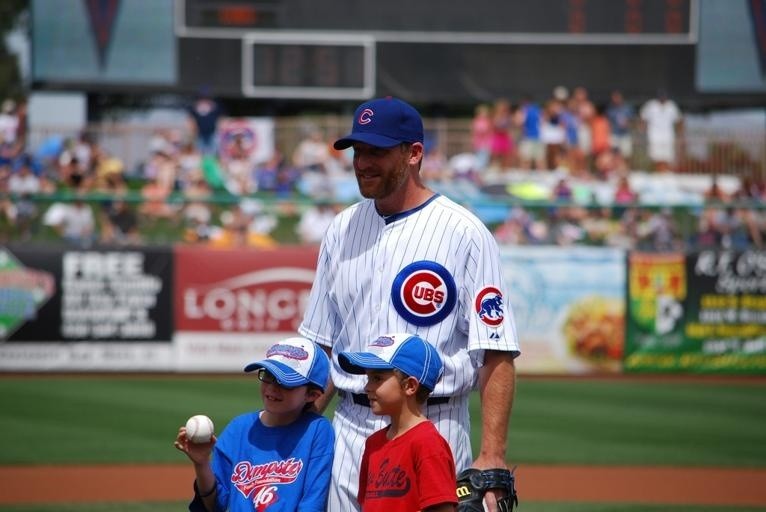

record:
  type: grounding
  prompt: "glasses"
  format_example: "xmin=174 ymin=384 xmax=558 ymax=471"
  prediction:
xmin=258 ymin=370 xmax=275 ymax=384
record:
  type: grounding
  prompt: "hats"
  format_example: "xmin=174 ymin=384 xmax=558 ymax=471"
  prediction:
xmin=338 ymin=333 xmax=443 ymax=391
xmin=244 ymin=338 xmax=329 ymax=391
xmin=334 ymin=96 xmax=424 ymax=150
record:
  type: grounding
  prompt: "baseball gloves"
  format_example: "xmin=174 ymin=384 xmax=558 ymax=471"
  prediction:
xmin=456 ymin=469 xmax=518 ymax=512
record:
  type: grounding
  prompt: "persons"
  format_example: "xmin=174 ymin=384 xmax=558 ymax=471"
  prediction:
xmin=2 ymin=85 xmax=365 ymax=248
xmin=336 ymin=331 xmax=458 ymax=511
xmin=174 ymin=337 xmax=331 ymax=512
xmin=418 ymin=85 xmax=766 ymax=247
xmin=297 ymin=97 xmax=520 ymax=512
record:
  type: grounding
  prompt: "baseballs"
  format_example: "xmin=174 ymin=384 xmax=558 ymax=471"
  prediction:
xmin=186 ymin=415 xmax=215 ymax=445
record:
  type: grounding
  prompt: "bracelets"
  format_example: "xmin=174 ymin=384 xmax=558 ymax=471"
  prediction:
xmin=193 ymin=478 xmax=218 ymax=498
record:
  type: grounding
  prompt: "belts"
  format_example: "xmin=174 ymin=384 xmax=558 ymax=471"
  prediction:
xmin=351 ymin=392 xmax=449 ymax=407
